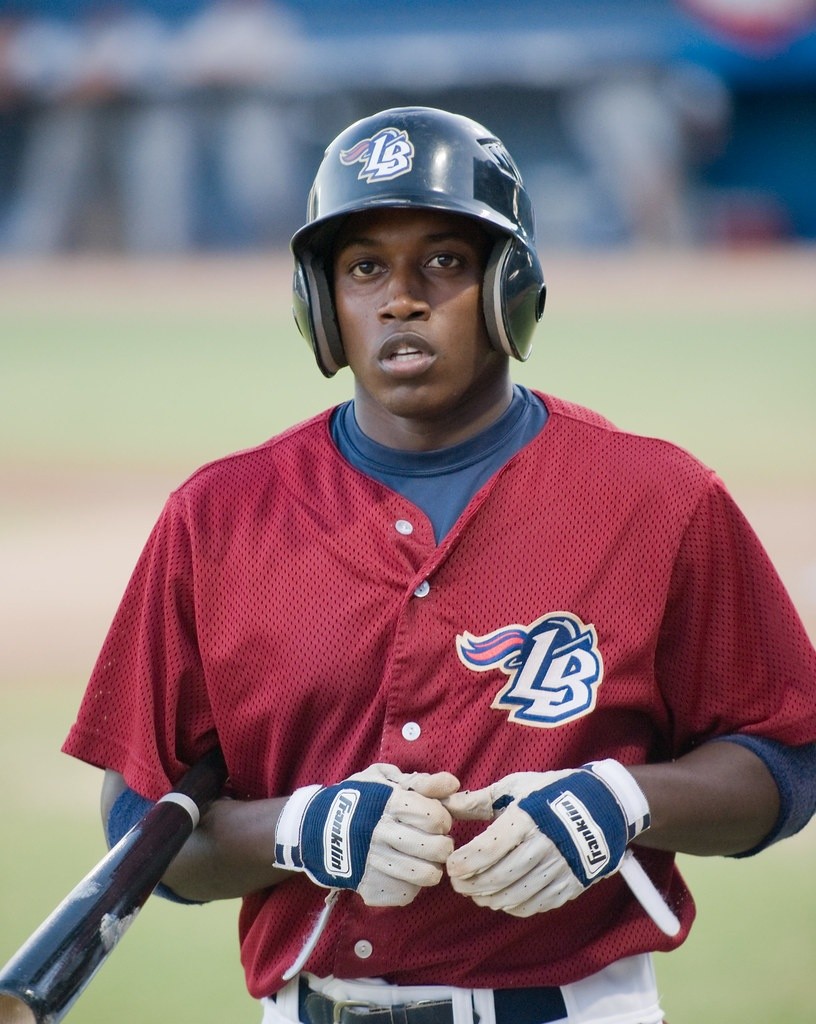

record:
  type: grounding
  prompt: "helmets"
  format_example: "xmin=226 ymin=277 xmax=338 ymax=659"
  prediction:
xmin=287 ymin=107 xmax=547 ymax=378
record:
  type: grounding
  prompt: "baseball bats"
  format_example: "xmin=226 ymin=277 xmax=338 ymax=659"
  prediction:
xmin=0 ymin=745 xmax=225 ymax=1024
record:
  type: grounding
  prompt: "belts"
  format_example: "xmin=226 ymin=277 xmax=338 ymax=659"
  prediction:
xmin=269 ymin=977 xmax=567 ymax=1023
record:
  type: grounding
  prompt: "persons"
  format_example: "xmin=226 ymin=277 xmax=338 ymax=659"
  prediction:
xmin=60 ymin=107 xmax=816 ymax=1024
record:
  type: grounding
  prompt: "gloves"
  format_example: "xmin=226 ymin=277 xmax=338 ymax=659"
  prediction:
xmin=441 ymin=758 xmax=652 ymax=917
xmin=272 ymin=763 xmax=461 ymax=907
